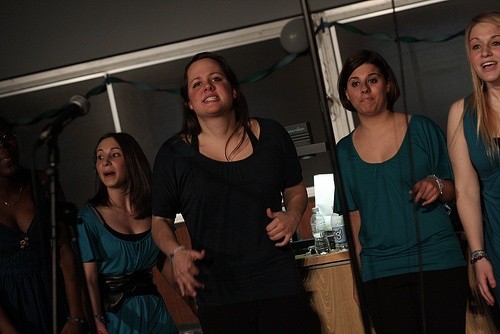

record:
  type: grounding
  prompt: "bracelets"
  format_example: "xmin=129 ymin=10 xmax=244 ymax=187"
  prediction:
xmin=93 ymin=311 xmax=106 ymax=323
xmin=427 ymin=175 xmax=445 ymax=195
xmin=168 ymin=245 xmax=186 ymax=264
xmin=67 ymin=317 xmax=86 ymax=323
xmin=470 ymin=250 xmax=489 ymax=264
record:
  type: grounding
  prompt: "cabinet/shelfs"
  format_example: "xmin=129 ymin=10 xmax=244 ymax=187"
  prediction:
xmin=295 ymin=250 xmax=500 ymax=334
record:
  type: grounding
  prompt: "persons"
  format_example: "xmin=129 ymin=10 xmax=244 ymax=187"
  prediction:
xmin=330 ymin=49 xmax=468 ymax=334
xmin=150 ymin=52 xmax=324 ymax=334
xmin=444 ymin=11 xmax=500 ymax=333
xmin=75 ymin=132 xmax=180 ymax=334
xmin=0 ymin=119 xmax=90 ymax=334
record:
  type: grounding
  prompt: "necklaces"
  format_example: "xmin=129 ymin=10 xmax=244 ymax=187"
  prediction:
xmin=1 ymin=181 xmax=23 ymax=206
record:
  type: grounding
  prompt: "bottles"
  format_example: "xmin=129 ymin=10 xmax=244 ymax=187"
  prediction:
xmin=330 ymin=205 xmax=349 ymax=252
xmin=310 ymin=207 xmax=331 ymax=255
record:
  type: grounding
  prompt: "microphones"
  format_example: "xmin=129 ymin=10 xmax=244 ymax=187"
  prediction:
xmin=35 ymin=94 xmax=92 ymax=146
